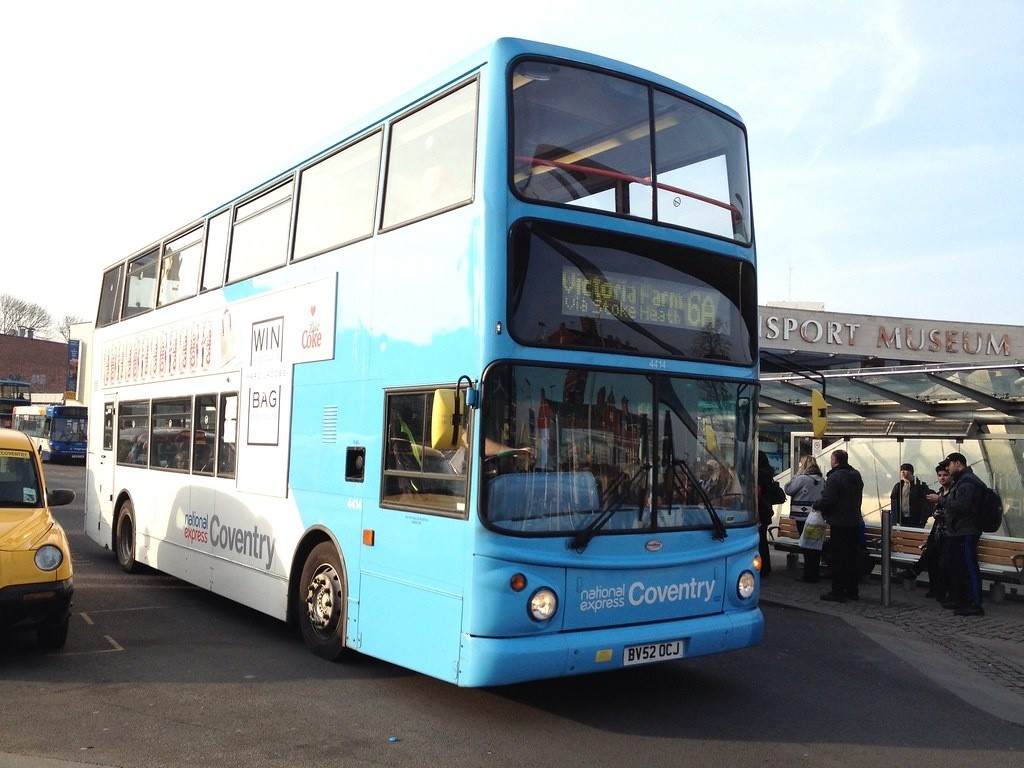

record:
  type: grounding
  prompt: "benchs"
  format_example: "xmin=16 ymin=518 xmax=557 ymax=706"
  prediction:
xmin=767 ymin=516 xmax=1024 ymax=604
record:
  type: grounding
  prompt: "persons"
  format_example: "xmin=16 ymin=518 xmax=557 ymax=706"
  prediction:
xmin=926 ymin=452 xmax=992 ymax=616
xmin=431 ymin=385 xmax=745 ymax=510
xmin=16 ymin=392 xmax=26 ymax=399
xmin=785 ymin=456 xmax=827 ymax=583
xmin=889 ymin=465 xmax=928 ymax=529
xmin=4 ymin=459 xmax=27 ymax=484
xmin=747 ymin=451 xmax=775 ymax=579
xmin=811 ymin=450 xmax=864 ymax=602
xmin=895 ymin=464 xmax=958 ymax=602
xmin=124 ymin=431 xmax=235 ymax=474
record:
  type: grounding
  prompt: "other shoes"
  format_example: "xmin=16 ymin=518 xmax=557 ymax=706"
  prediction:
xmin=820 ymin=592 xmax=845 ymax=601
xmin=953 ymin=605 xmax=985 ymax=616
xmin=942 ymin=599 xmax=963 ymax=609
xmin=897 ymin=570 xmax=916 ymax=579
xmin=844 ymin=591 xmax=860 ymax=600
xmin=925 ymin=589 xmax=937 ymax=598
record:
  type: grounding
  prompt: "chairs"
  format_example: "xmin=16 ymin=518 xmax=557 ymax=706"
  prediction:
xmin=118 ymin=430 xmax=235 ymax=474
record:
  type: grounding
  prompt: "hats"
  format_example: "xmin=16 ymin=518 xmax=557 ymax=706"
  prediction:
xmin=900 ymin=464 xmax=914 ymax=474
xmin=938 ymin=453 xmax=967 ymax=466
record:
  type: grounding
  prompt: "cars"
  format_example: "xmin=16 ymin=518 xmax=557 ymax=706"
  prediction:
xmin=0 ymin=426 xmax=77 ymax=651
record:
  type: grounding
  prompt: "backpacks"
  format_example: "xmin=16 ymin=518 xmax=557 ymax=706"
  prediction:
xmin=956 ymin=477 xmax=1003 ymax=533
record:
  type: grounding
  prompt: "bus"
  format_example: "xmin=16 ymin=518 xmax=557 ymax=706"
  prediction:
xmin=83 ymin=36 xmax=830 ymax=691
xmin=0 ymin=380 xmax=33 ymax=427
xmin=11 ymin=406 xmax=87 ymax=463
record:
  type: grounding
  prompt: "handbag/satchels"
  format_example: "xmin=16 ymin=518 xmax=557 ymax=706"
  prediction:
xmin=799 ymin=509 xmax=825 ymax=550
xmin=770 ymin=478 xmax=787 ymax=505
